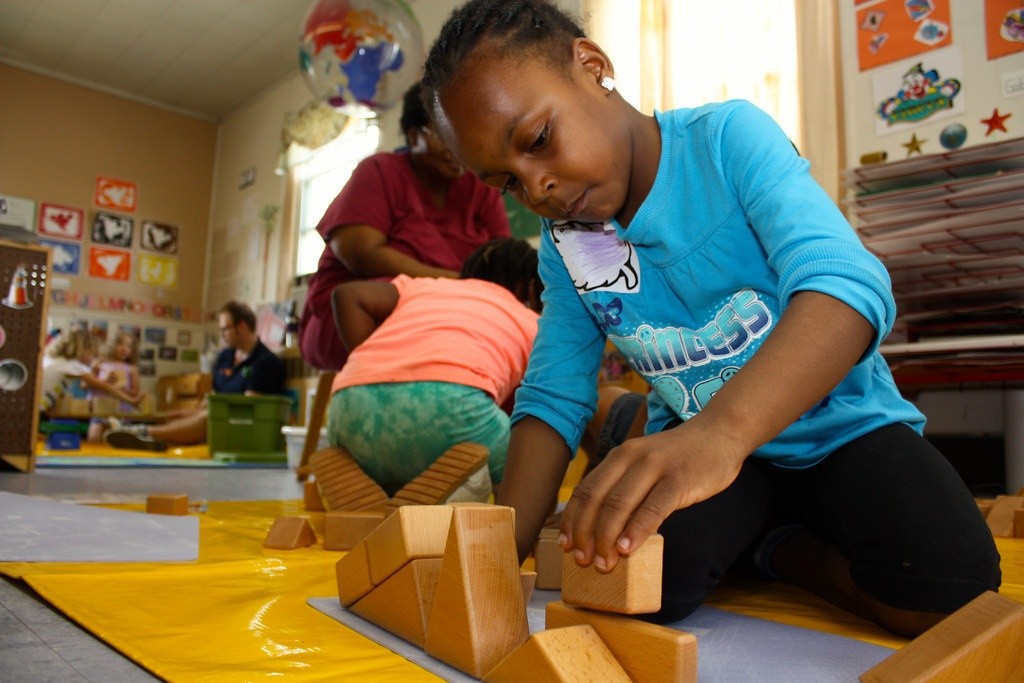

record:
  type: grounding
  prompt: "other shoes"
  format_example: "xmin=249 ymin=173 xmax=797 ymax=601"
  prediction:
xmin=104 ymin=425 xmax=168 ymax=452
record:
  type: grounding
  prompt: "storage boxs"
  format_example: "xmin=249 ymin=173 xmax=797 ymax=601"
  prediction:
xmin=207 ymin=394 xmax=291 ymax=463
xmin=282 ymin=422 xmax=327 ymax=475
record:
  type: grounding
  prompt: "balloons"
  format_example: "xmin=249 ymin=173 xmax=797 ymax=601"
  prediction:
xmin=299 ymin=0 xmax=425 ymax=118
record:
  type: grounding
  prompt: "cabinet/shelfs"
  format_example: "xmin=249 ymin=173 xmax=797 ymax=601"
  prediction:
xmin=157 ymin=372 xmax=214 ymax=412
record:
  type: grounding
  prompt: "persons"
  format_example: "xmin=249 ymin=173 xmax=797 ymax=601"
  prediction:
xmin=296 ymin=81 xmax=510 ymax=372
xmin=38 ymin=328 xmax=145 ymax=433
xmin=420 ymin=0 xmax=1002 ymax=637
xmin=104 ymin=301 xmax=294 ymax=453
xmin=309 ymin=236 xmax=545 ymax=521
xmin=80 ymin=332 xmax=139 ymax=442
